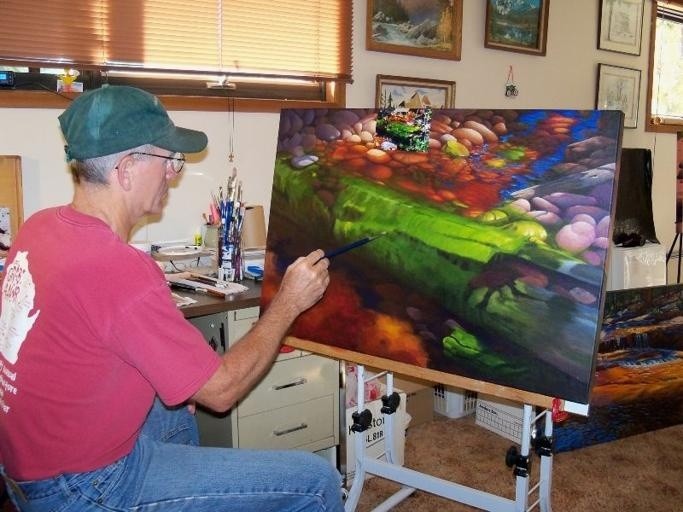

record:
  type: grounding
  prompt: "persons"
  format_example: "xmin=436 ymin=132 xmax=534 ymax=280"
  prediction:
xmin=0 ymin=82 xmax=343 ymax=512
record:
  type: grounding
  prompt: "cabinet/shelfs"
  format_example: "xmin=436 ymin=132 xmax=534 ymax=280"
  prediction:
xmin=163 ymin=266 xmax=348 ymax=498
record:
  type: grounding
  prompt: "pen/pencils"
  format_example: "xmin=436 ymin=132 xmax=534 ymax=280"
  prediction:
xmin=169 ymin=282 xmax=225 ymax=298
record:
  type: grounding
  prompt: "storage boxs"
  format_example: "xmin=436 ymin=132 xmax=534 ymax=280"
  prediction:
xmin=338 ymin=381 xmax=408 ymax=486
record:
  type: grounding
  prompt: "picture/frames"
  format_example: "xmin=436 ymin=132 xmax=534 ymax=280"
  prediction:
xmin=597 ymin=0 xmax=645 ymax=56
xmin=366 ymin=0 xmax=463 ymax=61
xmin=375 ymin=74 xmax=456 ymax=109
xmin=595 ymin=63 xmax=641 ymax=129
xmin=484 ymin=0 xmax=550 ymax=57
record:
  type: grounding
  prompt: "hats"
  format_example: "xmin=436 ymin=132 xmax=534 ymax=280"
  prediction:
xmin=58 ymin=84 xmax=208 ymax=161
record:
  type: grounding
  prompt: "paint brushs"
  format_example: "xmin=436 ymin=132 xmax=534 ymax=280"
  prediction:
xmin=185 ymin=274 xmax=228 ymax=288
xmin=209 ymin=168 xmax=245 ymax=242
xmin=321 ymin=225 xmax=397 ymax=260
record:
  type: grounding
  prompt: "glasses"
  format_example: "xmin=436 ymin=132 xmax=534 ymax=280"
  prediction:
xmin=115 ymin=151 xmax=185 ymax=173
